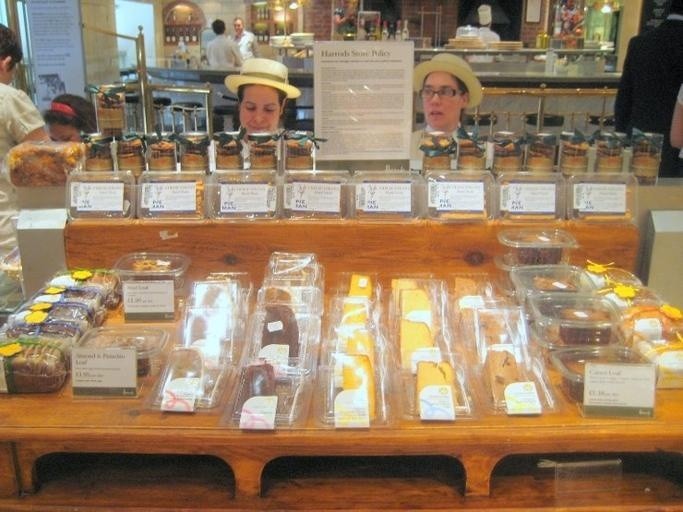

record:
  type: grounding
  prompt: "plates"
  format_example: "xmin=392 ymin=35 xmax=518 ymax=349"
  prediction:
xmin=287 ymin=32 xmax=315 ymax=46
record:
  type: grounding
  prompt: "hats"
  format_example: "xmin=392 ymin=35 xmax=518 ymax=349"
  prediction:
xmin=414 ymin=53 xmax=482 ymax=109
xmin=224 ymin=59 xmax=301 ymax=99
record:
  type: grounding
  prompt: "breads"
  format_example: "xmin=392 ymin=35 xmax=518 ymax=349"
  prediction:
xmin=1 ymin=236 xmax=683 ymax=418
xmin=9 ymin=142 xmax=84 ymax=186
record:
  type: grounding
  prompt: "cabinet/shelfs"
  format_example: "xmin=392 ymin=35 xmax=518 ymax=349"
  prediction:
xmin=0 ymin=218 xmax=682 ymax=511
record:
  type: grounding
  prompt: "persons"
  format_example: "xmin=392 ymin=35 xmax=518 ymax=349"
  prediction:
xmin=0 ymin=23 xmax=50 ymax=293
xmin=333 ymin=0 xmax=359 ymax=37
xmin=206 ymin=20 xmax=242 ymax=71
xmin=227 ymin=17 xmax=261 ymax=61
xmin=413 ymin=52 xmax=482 ymax=146
xmin=44 ymin=95 xmax=97 ymax=143
xmin=224 ymin=57 xmax=301 ymax=146
xmin=470 ymin=4 xmax=501 ymax=63
xmin=668 ymin=83 xmax=683 ymax=179
xmin=614 ymin=0 xmax=683 ymax=178
xmin=555 ymin=0 xmax=577 ymax=38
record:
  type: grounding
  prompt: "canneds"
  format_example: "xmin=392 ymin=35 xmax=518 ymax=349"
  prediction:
xmin=422 ymin=132 xmax=663 ymax=186
xmin=84 ymin=130 xmax=312 ymax=170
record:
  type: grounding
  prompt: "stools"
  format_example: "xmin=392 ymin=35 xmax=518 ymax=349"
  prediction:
xmin=125 ymin=92 xmax=237 ymax=134
xmin=460 ymin=113 xmax=615 ymax=139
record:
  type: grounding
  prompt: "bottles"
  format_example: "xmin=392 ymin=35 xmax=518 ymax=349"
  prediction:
xmin=355 ymin=18 xmax=410 ymax=43
xmin=168 ymin=27 xmax=197 ymax=44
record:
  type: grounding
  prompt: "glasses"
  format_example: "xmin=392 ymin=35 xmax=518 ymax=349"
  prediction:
xmin=419 ymin=87 xmax=459 ymax=98
